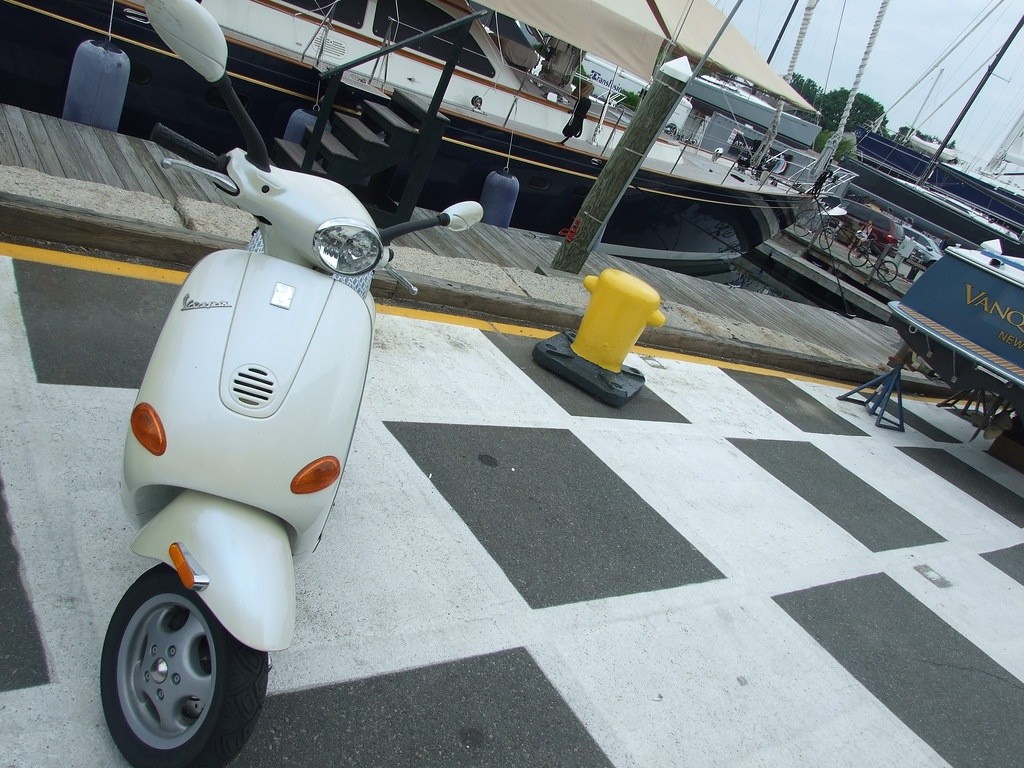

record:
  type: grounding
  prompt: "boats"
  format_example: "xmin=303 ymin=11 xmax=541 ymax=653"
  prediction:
xmin=887 ymin=236 xmax=1024 ymax=427
xmin=835 ymin=14 xmax=1024 ymax=260
xmin=909 ymin=136 xmax=957 ymax=161
xmin=1 ymin=0 xmax=1011 ymax=273
xmin=851 ymin=0 xmax=1023 ymax=233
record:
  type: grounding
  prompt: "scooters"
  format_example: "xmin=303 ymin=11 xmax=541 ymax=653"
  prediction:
xmin=100 ymin=1 xmax=486 ymax=768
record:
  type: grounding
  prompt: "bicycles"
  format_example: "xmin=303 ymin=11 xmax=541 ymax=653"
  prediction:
xmin=794 ymin=201 xmax=835 ymax=249
xmin=847 ymin=237 xmax=900 ymax=282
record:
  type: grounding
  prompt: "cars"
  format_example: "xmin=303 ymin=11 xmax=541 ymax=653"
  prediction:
xmin=901 ymin=225 xmax=943 ymax=267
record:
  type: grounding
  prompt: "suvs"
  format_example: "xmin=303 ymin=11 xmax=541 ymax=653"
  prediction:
xmin=837 ymin=198 xmax=904 ymax=255
